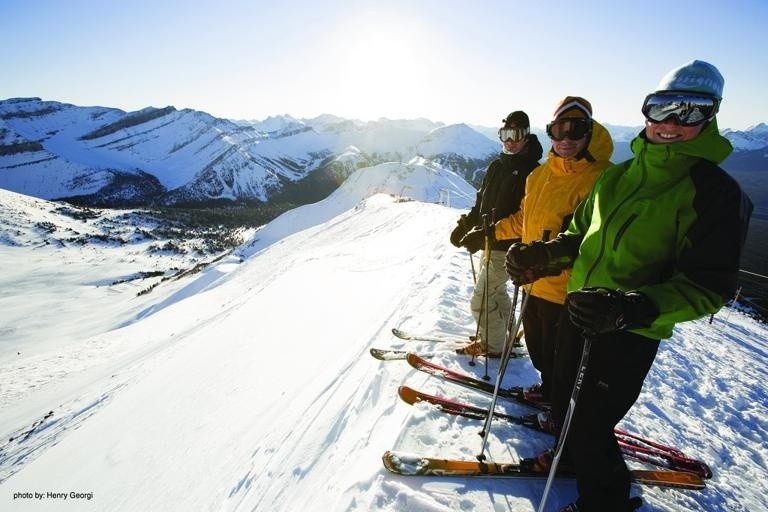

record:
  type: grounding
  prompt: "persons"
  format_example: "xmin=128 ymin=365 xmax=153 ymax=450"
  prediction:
xmin=505 ymin=59 xmax=753 ymax=512
xmin=450 ymin=111 xmax=543 ymax=356
xmin=459 ymin=96 xmax=617 ymax=436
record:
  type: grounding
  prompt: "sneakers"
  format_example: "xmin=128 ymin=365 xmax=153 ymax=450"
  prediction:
xmin=522 ymin=449 xmax=553 ymax=474
xmin=456 ymin=335 xmax=502 ymax=358
xmin=521 ymin=409 xmax=559 ymax=433
xmin=559 ymin=503 xmax=580 ymax=512
xmin=509 ymin=382 xmax=552 ymax=406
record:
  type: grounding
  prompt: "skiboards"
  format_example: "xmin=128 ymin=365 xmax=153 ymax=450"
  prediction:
xmin=369 ymin=328 xmax=530 ymax=361
xmin=382 ymin=449 xmax=713 ymax=511
xmin=398 ymin=353 xmax=714 ymax=479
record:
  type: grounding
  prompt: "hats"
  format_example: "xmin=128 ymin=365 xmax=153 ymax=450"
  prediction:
xmin=552 ymin=96 xmax=592 ymax=121
xmin=653 ymin=60 xmax=724 ymax=99
xmin=505 ymin=111 xmax=529 ymax=130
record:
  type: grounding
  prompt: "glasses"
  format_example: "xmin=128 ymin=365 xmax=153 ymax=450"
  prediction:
xmin=498 ymin=126 xmax=530 ymax=142
xmin=641 ymin=89 xmax=722 ymax=127
xmin=545 ymin=115 xmax=592 ymax=141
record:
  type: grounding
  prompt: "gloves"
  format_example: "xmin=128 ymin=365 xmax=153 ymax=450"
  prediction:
xmin=566 ymin=286 xmax=656 ymax=337
xmin=451 ymin=216 xmax=499 ymax=254
xmin=505 ymin=233 xmax=561 ymax=288
